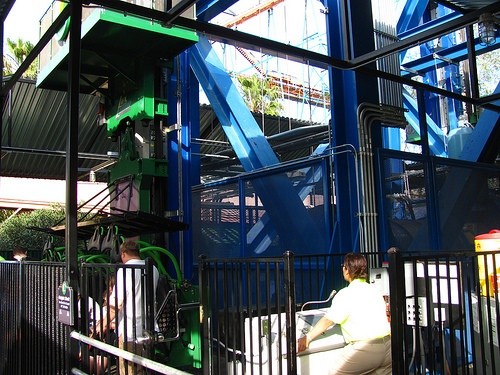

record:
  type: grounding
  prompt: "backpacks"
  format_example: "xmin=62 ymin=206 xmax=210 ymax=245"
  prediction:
xmin=156 ymin=271 xmax=186 ymax=340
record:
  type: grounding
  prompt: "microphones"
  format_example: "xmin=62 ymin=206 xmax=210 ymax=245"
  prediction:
xmin=301 ymin=290 xmax=336 ymax=311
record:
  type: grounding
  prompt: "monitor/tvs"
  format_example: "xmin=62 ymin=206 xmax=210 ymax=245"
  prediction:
xmin=298 ymin=313 xmax=324 ymax=327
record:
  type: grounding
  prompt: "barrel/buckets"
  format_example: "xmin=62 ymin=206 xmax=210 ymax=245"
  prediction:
xmin=475 ymin=229 xmax=500 ymax=297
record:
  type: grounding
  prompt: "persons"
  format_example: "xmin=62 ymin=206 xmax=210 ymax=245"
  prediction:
xmin=297 ymin=253 xmax=392 ymax=375
xmin=13 ymin=241 xmax=160 ymax=375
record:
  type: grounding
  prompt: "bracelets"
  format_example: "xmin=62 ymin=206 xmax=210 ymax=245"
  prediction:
xmin=305 ymin=334 xmax=312 ymax=342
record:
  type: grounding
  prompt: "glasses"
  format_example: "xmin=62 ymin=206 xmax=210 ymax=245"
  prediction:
xmin=340 ymin=263 xmax=346 ymax=268
